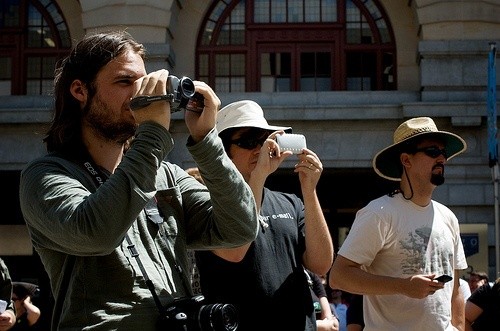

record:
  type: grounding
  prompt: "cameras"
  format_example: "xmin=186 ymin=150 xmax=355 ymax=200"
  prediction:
xmin=167 ymin=76 xmax=205 ymax=113
xmin=275 ymin=134 xmax=307 ymax=154
xmin=159 ymin=294 xmax=239 ymax=331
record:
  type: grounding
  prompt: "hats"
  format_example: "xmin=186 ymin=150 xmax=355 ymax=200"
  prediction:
xmin=373 ymin=116 xmax=466 ymax=181
xmin=216 ymin=100 xmax=293 ymax=135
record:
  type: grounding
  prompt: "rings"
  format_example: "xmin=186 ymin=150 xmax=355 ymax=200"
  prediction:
xmin=309 ymin=164 xmax=313 ymax=168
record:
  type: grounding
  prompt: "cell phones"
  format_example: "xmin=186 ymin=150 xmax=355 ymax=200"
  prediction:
xmin=433 ymin=275 xmax=453 ymax=283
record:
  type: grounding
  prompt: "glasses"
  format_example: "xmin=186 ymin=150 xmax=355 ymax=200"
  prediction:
xmin=12 ymin=296 xmax=27 ymax=303
xmin=228 ymin=134 xmax=270 ymax=150
xmin=412 ymin=145 xmax=449 ymax=161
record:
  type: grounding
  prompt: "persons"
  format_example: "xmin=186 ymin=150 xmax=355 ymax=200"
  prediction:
xmin=194 ymin=100 xmax=334 ymax=331
xmin=0 ymin=258 xmax=55 ymax=331
xmin=302 ymin=254 xmax=365 ymax=331
xmin=329 ymin=117 xmax=466 ymax=331
xmin=459 ymin=272 xmax=500 ymax=331
xmin=20 ymin=33 xmax=258 ymax=331
xmin=185 ymin=167 xmax=205 ymax=297
